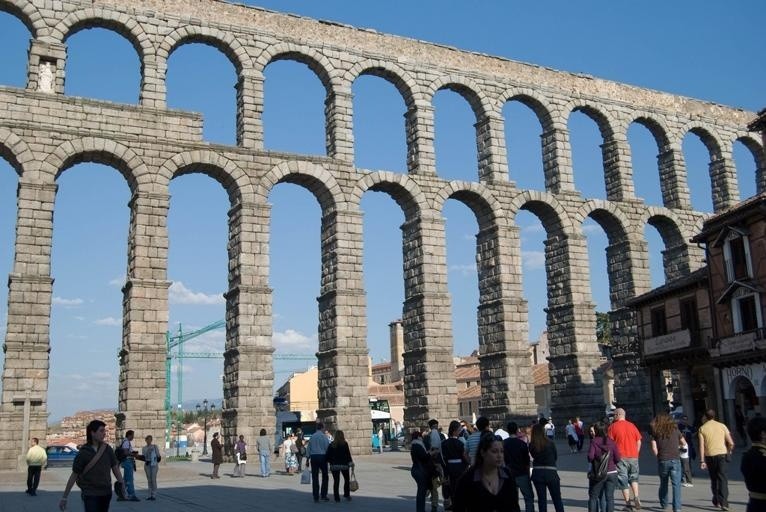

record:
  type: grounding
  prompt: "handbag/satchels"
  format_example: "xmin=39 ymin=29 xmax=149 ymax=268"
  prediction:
xmin=349 ymin=472 xmax=358 ymax=491
xmin=301 ymin=466 xmax=311 ymax=484
xmin=590 ymin=452 xmax=609 ymax=481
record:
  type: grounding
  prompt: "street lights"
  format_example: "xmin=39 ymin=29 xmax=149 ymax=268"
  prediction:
xmin=195 ymin=398 xmax=215 ymax=456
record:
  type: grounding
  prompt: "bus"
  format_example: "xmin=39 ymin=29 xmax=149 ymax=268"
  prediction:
xmin=275 ymin=409 xmax=397 ymax=452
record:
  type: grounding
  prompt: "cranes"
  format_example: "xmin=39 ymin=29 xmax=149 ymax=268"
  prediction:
xmin=176 ymin=321 xmax=317 ymax=437
xmin=166 ymin=318 xmax=226 ymax=450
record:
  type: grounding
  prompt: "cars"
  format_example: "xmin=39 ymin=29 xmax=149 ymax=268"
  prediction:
xmin=46 ymin=444 xmax=80 ymax=469
xmin=395 ymin=431 xmax=404 ymax=446
xmin=666 ymin=410 xmax=685 ymax=429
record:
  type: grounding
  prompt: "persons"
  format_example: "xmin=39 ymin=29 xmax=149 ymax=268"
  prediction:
xmin=411 ymin=418 xmax=564 ymax=511
xmin=377 ymin=426 xmax=383 ymax=454
xmin=120 ymin=430 xmax=141 ymax=501
xmin=650 ymin=411 xmax=687 ymax=511
xmin=566 ymin=416 xmax=584 ymax=453
xmin=234 ymin=435 xmax=247 ymax=478
xmin=257 ymin=429 xmax=273 ymax=477
xmin=754 ymin=409 xmax=761 ymax=416
xmin=283 ymin=422 xmax=355 ymax=504
xmin=698 ymin=408 xmax=734 ymax=511
xmin=211 ymin=432 xmax=224 ymax=479
xmin=586 ymin=408 xmax=642 ymax=511
xmin=677 ymin=414 xmax=698 ymax=488
xmin=142 ymin=435 xmax=161 ymax=500
xmin=59 ymin=420 xmax=126 ymax=512
xmin=26 ymin=438 xmax=47 ymax=496
xmin=741 ymin=416 xmax=766 ymax=512
xmin=733 ymin=408 xmax=747 ymax=447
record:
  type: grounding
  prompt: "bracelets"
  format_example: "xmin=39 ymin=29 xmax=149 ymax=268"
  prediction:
xmin=60 ymin=497 xmax=67 ymax=500
